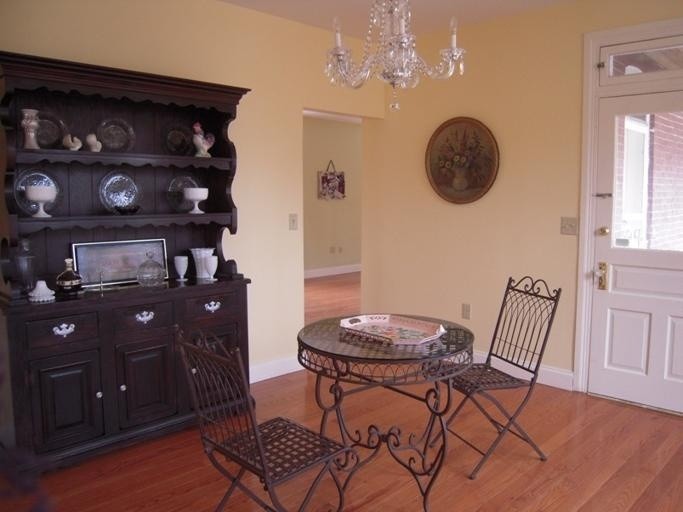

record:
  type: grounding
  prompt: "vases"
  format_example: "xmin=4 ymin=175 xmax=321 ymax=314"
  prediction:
xmin=21 ymin=108 xmax=41 ymax=150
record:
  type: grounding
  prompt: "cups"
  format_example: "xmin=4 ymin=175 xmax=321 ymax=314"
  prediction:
xmin=190 ymin=248 xmax=214 ymax=279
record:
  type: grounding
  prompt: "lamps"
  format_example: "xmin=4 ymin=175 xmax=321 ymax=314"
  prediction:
xmin=321 ymin=0 xmax=469 ymax=114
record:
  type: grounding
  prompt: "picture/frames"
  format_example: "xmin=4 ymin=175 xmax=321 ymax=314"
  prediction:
xmin=425 ymin=116 xmax=500 ymax=205
xmin=72 ymin=238 xmax=169 ymax=289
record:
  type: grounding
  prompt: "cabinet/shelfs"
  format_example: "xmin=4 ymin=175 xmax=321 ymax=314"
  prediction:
xmin=1 ymin=50 xmax=253 ymax=491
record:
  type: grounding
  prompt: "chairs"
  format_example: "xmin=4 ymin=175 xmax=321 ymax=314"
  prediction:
xmin=432 ymin=276 xmax=564 ymax=480
xmin=171 ymin=328 xmax=351 ymax=512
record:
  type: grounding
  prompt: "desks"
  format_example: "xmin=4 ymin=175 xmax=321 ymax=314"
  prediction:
xmin=295 ymin=313 xmax=474 ymax=512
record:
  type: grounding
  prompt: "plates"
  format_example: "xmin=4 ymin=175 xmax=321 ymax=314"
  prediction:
xmin=99 ymin=170 xmax=140 ymax=213
xmin=168 ymin=128 xmax=192 ymax=154
xmin=36 ymin=114 xmax=66 ymax=149
xmin=14 ymin=169 xmax=63 ymax=216
xmin=166 ymin=174 xmax=199 ymax=211
xmin=98 ymin=118 xmax=136 ymax=152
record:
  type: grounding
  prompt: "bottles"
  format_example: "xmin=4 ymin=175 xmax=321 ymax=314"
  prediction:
xmin=136 ymin=252 xmax=164 ymax=288
xmin=56 ymin=259 xmax=82 ymax=292
xmin=17 ymin=241 xmax=39 ymax=291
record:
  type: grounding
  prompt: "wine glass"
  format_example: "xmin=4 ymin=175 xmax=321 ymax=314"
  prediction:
xmin=175 ymin=256 xmax=188 ymax=281
xmin=24 ymin=185 xmax=53 ymax=217
xmin=205 ymin=256 xmax=218 ymax=280
xmin=184 ymin=188 xmax=208 ymax=214
